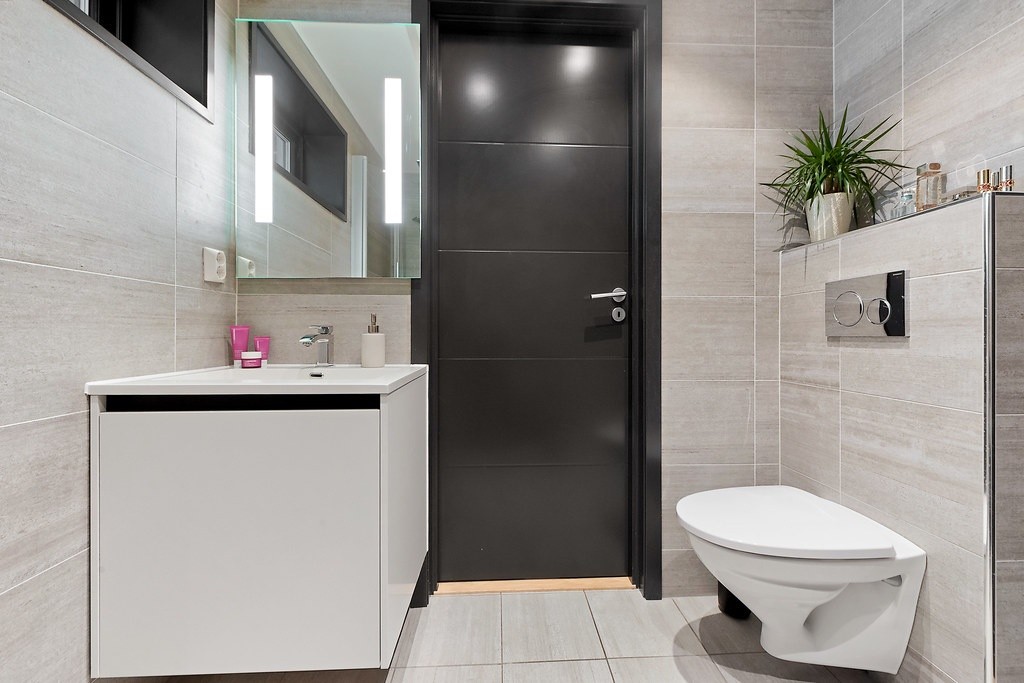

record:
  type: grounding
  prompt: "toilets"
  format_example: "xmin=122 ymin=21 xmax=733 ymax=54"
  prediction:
xmin=676 ymin=484 xmax=927 ymax=676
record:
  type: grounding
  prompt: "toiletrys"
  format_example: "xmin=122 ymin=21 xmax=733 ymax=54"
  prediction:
xmin=230 ymin=326 xmax=271 ymax=369
xmin=361 ymin=313 xmax=386 ymax=368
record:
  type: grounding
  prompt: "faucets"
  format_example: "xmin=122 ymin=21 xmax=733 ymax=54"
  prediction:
xmin=298 ymin=323 xmax=335 ymax=368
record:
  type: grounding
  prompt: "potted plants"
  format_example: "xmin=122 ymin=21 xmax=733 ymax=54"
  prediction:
xmin=759 ymin=103 xmax=914 ymax=243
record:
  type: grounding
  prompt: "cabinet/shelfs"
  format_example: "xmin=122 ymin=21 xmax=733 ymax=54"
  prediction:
xmin=88 ymin=372 xmax=432 ymax=682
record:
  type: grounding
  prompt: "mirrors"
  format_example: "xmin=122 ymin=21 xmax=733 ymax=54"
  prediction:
xmin=234 ymin=18 xmax=422 ymax=277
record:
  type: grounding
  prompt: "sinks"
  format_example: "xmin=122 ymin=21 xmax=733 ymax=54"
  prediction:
xmin=112 ymin=369 xmax=410 ymax=384
